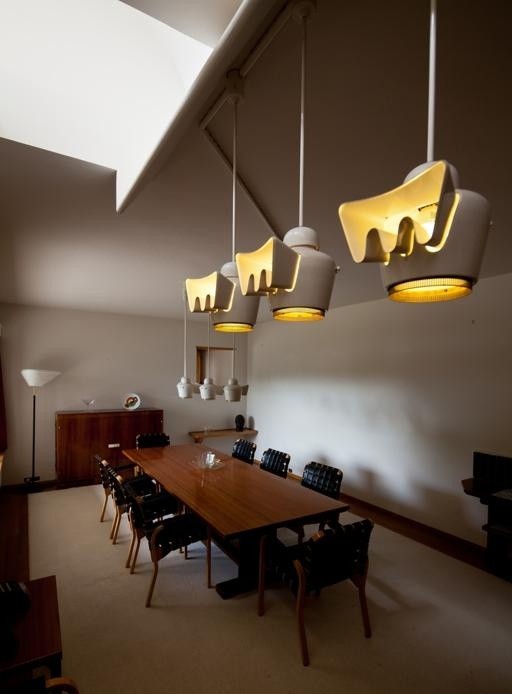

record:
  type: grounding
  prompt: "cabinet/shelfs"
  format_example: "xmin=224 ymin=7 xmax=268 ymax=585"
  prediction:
xmin=54 ymin=408 xmax=164 ymax=485
xmin=461 ymin=479 xmax=512 ymax=583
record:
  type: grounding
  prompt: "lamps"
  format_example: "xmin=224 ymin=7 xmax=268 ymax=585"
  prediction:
xmin=338 ymin=0 xmax=492 ymax=303
xmin=199 ymin=309 xmax=218 ymax=400
xmin=185 ymin=67 xmax=262 ymax=334
xmin=14 ymin=369 xmax=60 ymax=494
xmin=236 ymin=7 xmax=340 ymax=324
xmin=224 ymin=331 xmax=241 ymax=403
xmin=177 ymin=292 xmax=195 ymax=399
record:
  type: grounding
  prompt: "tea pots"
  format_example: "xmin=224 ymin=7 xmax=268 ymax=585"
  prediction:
xmin=195 ymin=448 xmax=216 ymax=468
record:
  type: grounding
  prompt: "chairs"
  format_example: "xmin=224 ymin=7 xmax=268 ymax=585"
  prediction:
xmin=264 ymin=518 xmax=374 ymax=668
xmin=95 ymin=453 xmax=148 ymax=538
xmin=112 ymin=475 xmax=185 ymax=568
xmin=299 ymin=461 xmax=343 ymax=499
xmin=258 ymin=448 xmax=290 ymax=479
xmin=123 ymin=482 xmax=212 ymax=609
xmin=231 ymin=439 xmax=255 ymax=462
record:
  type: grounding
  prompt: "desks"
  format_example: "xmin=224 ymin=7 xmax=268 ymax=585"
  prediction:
xmin=121 ymin=443 xmax=349 ymax=601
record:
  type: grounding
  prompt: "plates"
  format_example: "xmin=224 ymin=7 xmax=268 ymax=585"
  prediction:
xmin=191 ymin=460 xmax=227 ymax=471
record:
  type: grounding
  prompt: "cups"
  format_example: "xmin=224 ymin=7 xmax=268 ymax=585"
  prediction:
xmin=81 ymin=399 xmax=96 ymax=410
